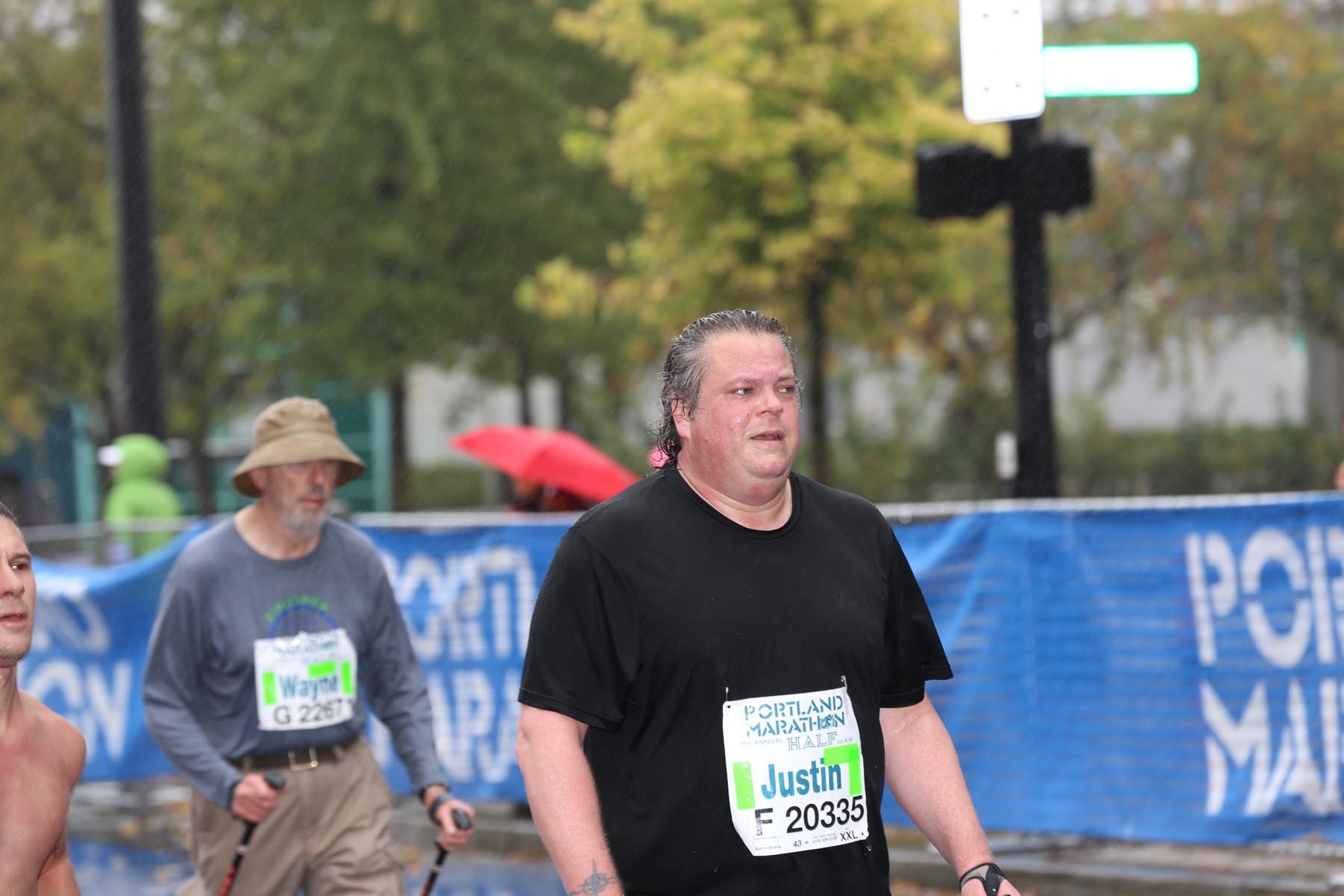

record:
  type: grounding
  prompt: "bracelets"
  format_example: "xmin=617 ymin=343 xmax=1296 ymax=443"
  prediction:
xmin=429 ymin=793 xmax=459 ymax=824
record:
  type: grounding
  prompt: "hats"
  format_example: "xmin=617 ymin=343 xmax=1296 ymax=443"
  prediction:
xmin=232 ymin=396 xmax=365 ymax=499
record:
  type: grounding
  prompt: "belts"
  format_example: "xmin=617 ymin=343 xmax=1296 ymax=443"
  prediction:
xmin=230 ymin=735 xmax=354 ymax=773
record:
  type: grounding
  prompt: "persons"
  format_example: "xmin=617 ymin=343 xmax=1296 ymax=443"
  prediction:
xmin=0 ymin=394 xmax=594 ymax=896
xmin=516 ymin=311 xmax=1023 ymax=895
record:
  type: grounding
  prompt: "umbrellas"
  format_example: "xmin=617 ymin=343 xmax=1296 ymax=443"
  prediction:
xmin=453 ymin=422 xmax=642 ymax=507
